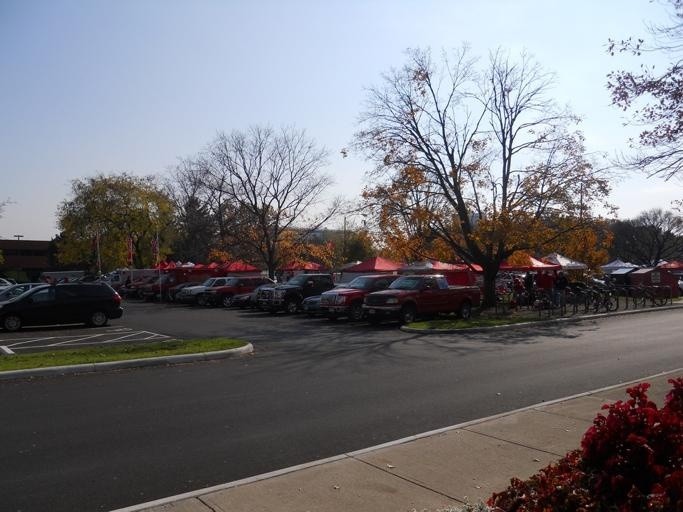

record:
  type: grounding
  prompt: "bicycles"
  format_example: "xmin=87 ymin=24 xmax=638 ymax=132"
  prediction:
xmin=633 ymin=283 xmax=667 ymax=306
xmin=585 ymin=288 xmax=619 ymax=312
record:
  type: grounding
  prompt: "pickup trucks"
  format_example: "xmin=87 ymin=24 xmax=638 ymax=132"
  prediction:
xmin=362 ymin=275 xmax=480 ymax=323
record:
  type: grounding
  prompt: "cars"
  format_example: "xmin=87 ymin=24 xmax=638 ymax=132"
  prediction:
xmin=118 ymin=277 xmax=202 ymax=303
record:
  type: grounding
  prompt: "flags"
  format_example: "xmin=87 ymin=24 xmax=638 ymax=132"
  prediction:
xmin=151 ymin=224 xmax=160 ymax=266
xmin=95 ymin=227 xmax=103 ymax=266
xmin=126 ymin=237 xmax=136 ymax=267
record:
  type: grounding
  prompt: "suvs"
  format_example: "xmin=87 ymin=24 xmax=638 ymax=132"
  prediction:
xmin=176 ymin=274 xmax=403 ymax=322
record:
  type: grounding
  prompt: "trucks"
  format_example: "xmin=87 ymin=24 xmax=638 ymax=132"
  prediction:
xmin=105 ymin=268 xmax=164 ymax=293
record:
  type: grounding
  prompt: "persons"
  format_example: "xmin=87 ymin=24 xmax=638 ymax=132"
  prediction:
xmin=512 ymin=270 xmax=583 ymax=310
xmin=125 ymin=276 xmax=132 ymax=288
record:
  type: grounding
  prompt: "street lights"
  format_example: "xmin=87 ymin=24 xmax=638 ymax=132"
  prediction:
xmin=14 ymin=235 xmax=23 ymax=283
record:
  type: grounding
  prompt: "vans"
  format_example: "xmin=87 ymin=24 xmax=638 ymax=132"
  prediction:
xmin=0 ymin=278 xmax=124 ymax=331
